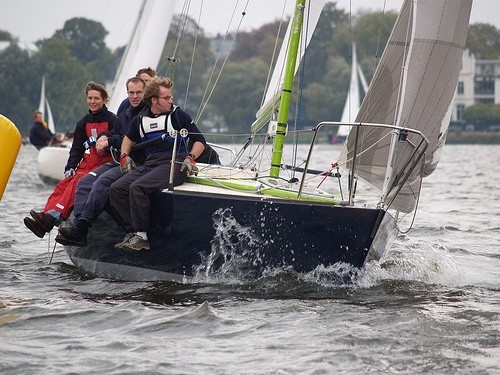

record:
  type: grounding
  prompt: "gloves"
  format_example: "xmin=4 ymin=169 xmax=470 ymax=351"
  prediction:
xmin=64 ymin=168 xmax=76 ymax=179
xmin=180 ymin=154 xmax=198 ymax=176
xmin=120 ymin=153 xmax=135 ymax=174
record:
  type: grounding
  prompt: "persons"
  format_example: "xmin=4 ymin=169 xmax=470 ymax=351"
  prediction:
xmin=54 ymin=66 xmax=157 ymax=245
xmin=29 ymin=111 xmax=54 ymax=150
xmin=110 ymin=77 xmax=206 ymax=252
xmin=23 ymin=80 xmax=118 ymax=239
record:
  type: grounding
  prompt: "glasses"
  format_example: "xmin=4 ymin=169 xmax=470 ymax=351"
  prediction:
xmin=154 ymin=95 xmax=173 ymax=100
xmin=127 ymin=91 xmax=143 ymax=96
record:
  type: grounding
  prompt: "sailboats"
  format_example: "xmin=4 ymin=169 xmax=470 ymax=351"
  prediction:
xmin=36 ymin=0 xmax=474 ymax=297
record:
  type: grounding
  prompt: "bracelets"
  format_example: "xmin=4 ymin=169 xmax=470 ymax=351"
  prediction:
xmin=187 ymin=153 xmax=195 ymax=158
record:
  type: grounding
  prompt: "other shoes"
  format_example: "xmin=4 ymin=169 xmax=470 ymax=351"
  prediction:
xmin=55 ymin=234 xmax=87 ymax=247
xmin=58 ymin=225 xmax=86 ymax=241
xmin=24 ymin=216 xmax=46 ymax=238
xmin=30 ymin=210 xmax=55 ymax=233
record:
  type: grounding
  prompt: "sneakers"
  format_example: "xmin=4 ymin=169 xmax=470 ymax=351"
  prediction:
xmin=114 ymin=233 xmax=136 ymax=252
xmin=123 ymin=235 xmax=150 ymax=250
